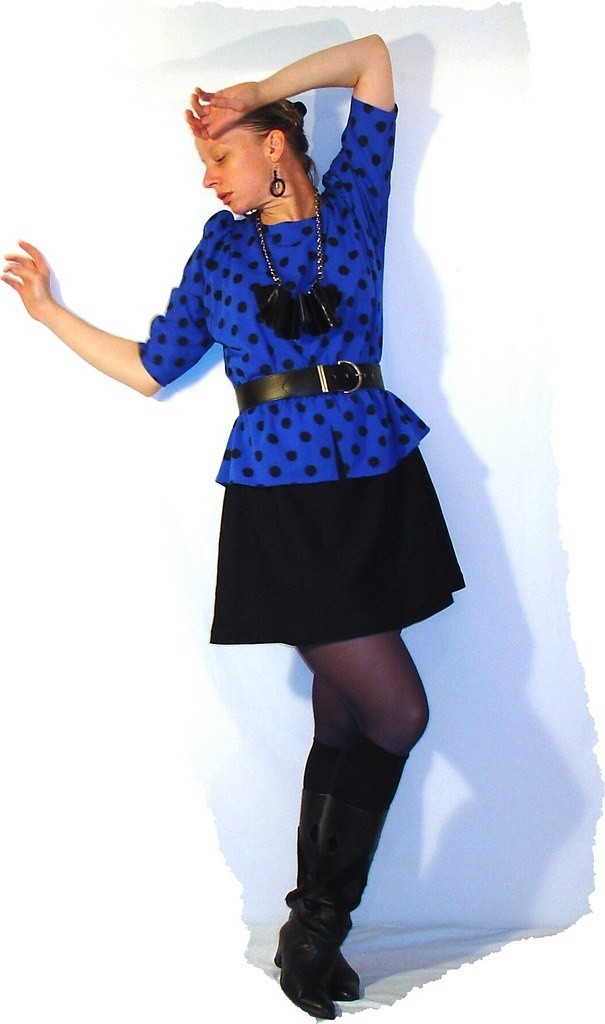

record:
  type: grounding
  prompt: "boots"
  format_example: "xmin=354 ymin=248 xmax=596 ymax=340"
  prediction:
xmin=274 ymin=732 xmax=361 ymax=1002
xmin=274 ymin=734 xmax=411 ymax=1020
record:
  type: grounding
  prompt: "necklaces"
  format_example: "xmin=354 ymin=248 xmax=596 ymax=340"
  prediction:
xmin=255 ymin=190 xmax=341 ymax=343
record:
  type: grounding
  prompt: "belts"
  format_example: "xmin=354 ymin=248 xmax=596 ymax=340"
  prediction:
xmin=235 ymin=359 xmax=386 ymax=414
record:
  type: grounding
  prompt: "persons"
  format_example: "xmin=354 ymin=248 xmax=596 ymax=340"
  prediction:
xmin=0 ymin=34 xmax=468 ymax=1019
